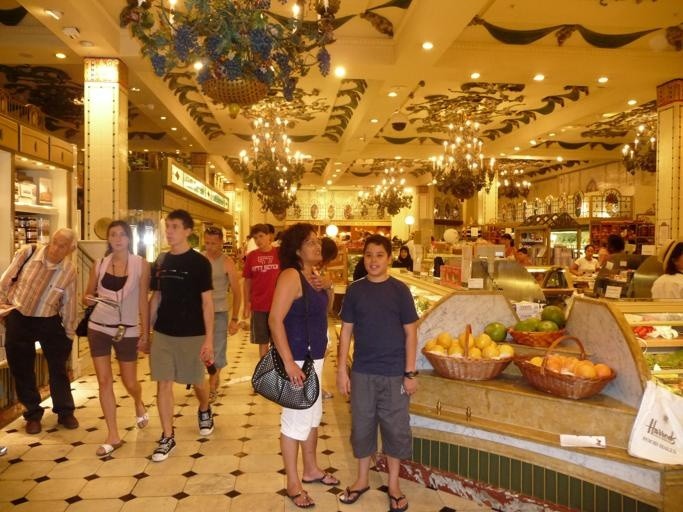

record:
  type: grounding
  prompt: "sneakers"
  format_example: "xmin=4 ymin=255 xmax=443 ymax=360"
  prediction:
xmin=152 ymin=436 xmax=176 ymax=462
xmin=198 ymin=405 xmax=214 ymax=435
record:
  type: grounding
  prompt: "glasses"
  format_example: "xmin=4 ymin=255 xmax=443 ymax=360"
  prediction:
xmin=302 ymin=238 xmax=324 ymax=247
xmin=205 ymin=227 xmax=222 ymax=235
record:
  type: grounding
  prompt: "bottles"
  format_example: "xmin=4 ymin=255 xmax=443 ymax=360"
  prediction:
xmin=13 ymin=215 xmax=49 ymax=244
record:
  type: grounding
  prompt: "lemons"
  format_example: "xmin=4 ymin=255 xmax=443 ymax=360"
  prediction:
xmin=424 ymin=334 xmax=501 ymax=359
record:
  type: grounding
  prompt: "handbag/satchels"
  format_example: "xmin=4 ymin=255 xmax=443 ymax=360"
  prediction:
xmin=76 ymin=299 xmax=97 ymax=337
xmin=251 ymin=346 xmax=320 ymax=409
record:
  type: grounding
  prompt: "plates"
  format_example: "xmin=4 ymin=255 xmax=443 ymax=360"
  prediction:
xmin=93 ymin=217 xmax=113 ymax=239
xmin=428 ymin=295 xmax=442 ymax=301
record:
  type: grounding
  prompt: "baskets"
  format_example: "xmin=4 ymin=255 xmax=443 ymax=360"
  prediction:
xmin=421 ymin=323 xmax=513 ymax=383
xmin=512 ymin=336 xmax=612 ymax=401
xmin=505 ymin=326 xmax=567 ymax=348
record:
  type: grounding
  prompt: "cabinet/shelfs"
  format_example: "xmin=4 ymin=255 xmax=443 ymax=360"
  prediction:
xmin=336 ymin=268 xmax=523 ymax=378
xmin=565 ymin=296 xmax=683 ymax=410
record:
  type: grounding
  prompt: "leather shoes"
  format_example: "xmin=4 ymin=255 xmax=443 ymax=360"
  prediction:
xmin=58 ymin=415 xmax=79 ymax=429
xmin=0 ymin=447 xmax=8 ymax=456
xmin=26 ymin=421 xmax=41 ymax=434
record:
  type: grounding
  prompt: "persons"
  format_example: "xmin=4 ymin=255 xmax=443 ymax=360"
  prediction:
xmin=241 ymin=222 xmax=413 ymax=277
xmin=196 ymin=221 xmax=241 ymax=405
xmin=134 ymin=209 xmax=216 ymax=462
xmin=266 ymin=222 xmax=341 ymax=510
xmin=502 ymin=233 xmax=533 ymax=265
xmin=652 ymin=243 xmax=683 ymax=299
xmin=433 ymin=255 xmax=444 ymax=277
xmin=569 ymin=233 xmax=625 ymax=277
xmin=0 ymin=225 xmax=79 ymax=434
xmin=335 ymin=234 xmax=419 ymax=512
xmin=81 ymin=219 xmax=152 ymax=457
xmin=244 ymin=222 xmax=285 ymax=361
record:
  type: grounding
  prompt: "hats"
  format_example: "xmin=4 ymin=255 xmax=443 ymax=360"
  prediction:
xmin=657 ymin=238 xmax=682 ymax=270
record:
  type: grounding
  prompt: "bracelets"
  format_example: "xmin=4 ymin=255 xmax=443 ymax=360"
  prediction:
xmin=230 ymin=317 xmax=238 ymax=321
xmin=403 ymin=371 xmax=418 ymax=378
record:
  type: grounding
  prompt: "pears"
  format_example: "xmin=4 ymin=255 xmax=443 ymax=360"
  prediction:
xmin=515 ymin=304 xmax=562 ymax=331
xmin=483 ymin=323 xmax=506 ymax=342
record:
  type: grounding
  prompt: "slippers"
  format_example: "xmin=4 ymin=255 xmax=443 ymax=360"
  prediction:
xmin=339 ymin=486 xmax=370 ymax=504
xmin=288 ymin=489 xmax=316 ymax=509
xmin=302 ymin=472 xmax=340 ymax=486
xmin=135 ymin=402 xmax=149 ymax=429
xmin=389 ymin=492 xmax=409 ymax=512
xmin=96 ymin=441 xmax=125 ymax=457
